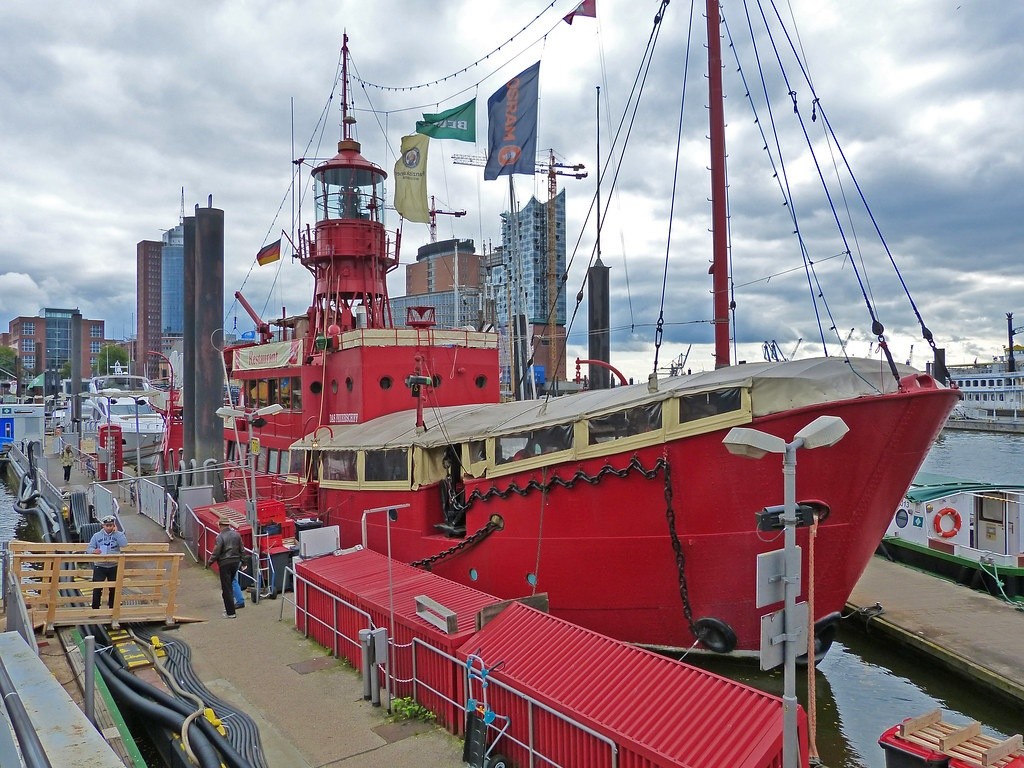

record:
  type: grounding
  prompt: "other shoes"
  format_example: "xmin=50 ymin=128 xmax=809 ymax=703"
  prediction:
xmin=234 ymin=602 xmax=245 ymax=609
xmin=222 ymin=612 xmax=236 ymax=619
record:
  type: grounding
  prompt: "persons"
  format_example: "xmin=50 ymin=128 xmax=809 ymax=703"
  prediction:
xmin=10 ymin=381 xmax=17 ymax=395
xmin=206 ymin=518 xmax=248 ymax=620
xmin=86 ymin=515 xmax=128 ymax=619
xmin=60 ymin=447 xmax=74 ymax=484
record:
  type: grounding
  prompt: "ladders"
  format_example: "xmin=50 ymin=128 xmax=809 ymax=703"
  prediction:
xmin=210 ymin=505 xmax=260 ymax=529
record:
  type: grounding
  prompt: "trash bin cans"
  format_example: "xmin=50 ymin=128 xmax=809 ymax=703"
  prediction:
xmin=263 ymin=546 xmax=291 ymax=593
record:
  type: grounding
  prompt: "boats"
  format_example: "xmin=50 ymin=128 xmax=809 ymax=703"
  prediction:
xmin=52 ymin=370 xmax=167 ymax=473
xmin=99 ymin=2 xmax=964 ymax=768
xmin=881 ymin=470 xmax=1024 ymax=597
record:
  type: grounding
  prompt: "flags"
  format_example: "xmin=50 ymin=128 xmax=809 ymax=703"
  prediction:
xmin=563 ymin=0 xmax=596 ymax=25
xmin=257 ymin=238 xmax=281 ymax=266
xmin=484 ymin=59 xmax=541 ymax=181
xmin=415 ymin=97 xmax=476 ymax=144
xmin=393 ymin=133 xmax=432 ymax=226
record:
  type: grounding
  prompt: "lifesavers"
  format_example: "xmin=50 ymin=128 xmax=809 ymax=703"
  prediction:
xmin=934 ymin=507 xmax=962 ymax=538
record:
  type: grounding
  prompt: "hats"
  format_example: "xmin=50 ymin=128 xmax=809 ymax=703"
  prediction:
xmin=100 ymin=515 xmax=116 ymax=524
xmin=219 ymin=516 xmax=231 ymax=525
